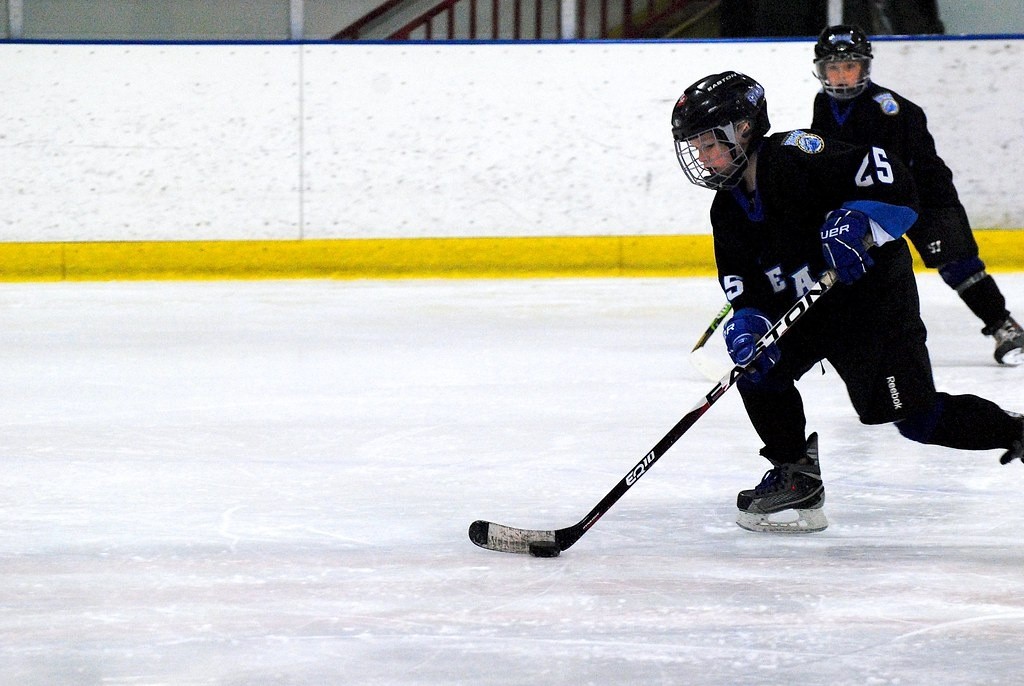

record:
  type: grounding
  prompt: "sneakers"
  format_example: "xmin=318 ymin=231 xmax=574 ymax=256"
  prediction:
xmin=994 ymin=316 xmax=1024 ymax=365
xmin=736 ymin=431 xmax=828 ymax=534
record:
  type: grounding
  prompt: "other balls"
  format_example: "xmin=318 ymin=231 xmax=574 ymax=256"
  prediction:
xmin=529 ymin=541 xmax=562 ymax=557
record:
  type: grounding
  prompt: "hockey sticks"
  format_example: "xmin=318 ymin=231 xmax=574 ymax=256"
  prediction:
xmin=469 ymin=273 xmax=840 ymax=557
xmin=690 ymin=300 xmax=733 ymax=382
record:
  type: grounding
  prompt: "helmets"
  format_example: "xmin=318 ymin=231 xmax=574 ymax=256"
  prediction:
xmin=814 ymin=24 xmax=874 ymax=100
xmin=671 ymin=71 xmax=770 ymax=192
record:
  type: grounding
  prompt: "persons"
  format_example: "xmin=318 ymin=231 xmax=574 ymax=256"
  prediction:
xmin=672 ymin=71 xmax=1024 ymax=534
xmin=810 ymin=26 xmax=1024 ymax=367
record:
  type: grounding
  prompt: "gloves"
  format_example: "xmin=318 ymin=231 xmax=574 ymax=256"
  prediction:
xmin=820 ymin=209 xmax=872 ymax=284
xmin=723 ymin=308 xmax=781 ymax=383
